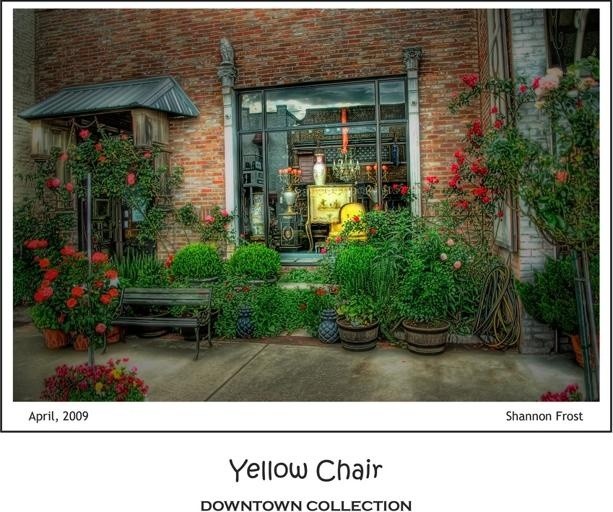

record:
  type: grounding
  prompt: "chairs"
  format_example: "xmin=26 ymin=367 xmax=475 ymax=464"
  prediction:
xmin=327 ymin=202 xmax=368 ymax=242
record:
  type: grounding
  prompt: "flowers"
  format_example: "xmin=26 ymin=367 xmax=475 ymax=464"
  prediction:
xmin=365 ymin=162 xmax=388 ymax=186
xmin=22 ymin=238 xmax=122 ymax=351
xmin=277 ymin=167 xmax=302 ymax=188
xmin=222 ymin=274 xmax=257 ymax=309
xmin=38 ymin=355 xmax=148 ymax=401
xmin=299 ymin=284 xmax=337 ymax=313
xmin=538 ymin=383 xmax=582 ymax=401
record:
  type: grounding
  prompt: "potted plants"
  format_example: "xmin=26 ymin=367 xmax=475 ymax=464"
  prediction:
xmin=333 ymin=244 xmax=454 ymax=354
xmin=130 ymin=271 xmax=222 ymax=341
xmin=512 ymin=253 xmax=599 ymax=370
xmin=171 ymin=241 xmax=282 ymax=290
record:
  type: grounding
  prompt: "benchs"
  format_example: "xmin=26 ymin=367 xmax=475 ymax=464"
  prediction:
xmin=99 ymin=287 xmax=212 ymax=361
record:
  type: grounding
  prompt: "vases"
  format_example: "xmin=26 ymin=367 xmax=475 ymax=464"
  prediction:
xmin=369 ymin=184 xmax=378 ymax=204
xmin=316 ymin=308 xmax=340 ymax=344
xmin=284 ymin=187 xmax=297 ymax=214
xmin=312 ymin=154 xmax=326 ymax=185
xmin=235 ymin=306 xmax=256 ymax=338
